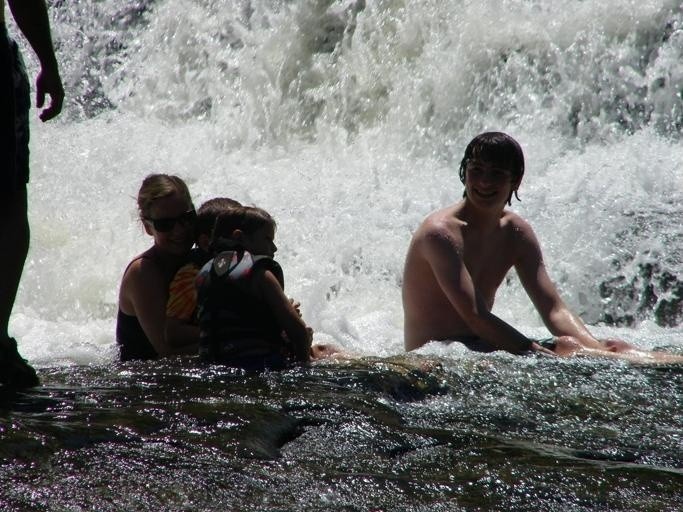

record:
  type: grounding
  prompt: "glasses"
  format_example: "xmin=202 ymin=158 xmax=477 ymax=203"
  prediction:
xmin=144 ymin=204 xmax=197 ymax=232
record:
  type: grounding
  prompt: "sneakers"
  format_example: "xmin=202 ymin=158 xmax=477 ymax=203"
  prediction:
xmin=0 ymin=337 xmax=39 ymax=388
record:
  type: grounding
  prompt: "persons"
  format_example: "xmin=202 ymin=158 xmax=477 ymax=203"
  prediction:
xmin=191 ymin=204 xmax=349 ymax=367
xmin=401 ymin=130 xmax=683 ymax=366
xmin=0 ymin=0 xmax=65 ymax=387
xmin=165 ymin=197 xmax=242 ymax=355
xmin=114 ymin=173 xmax=199 ymax=364
